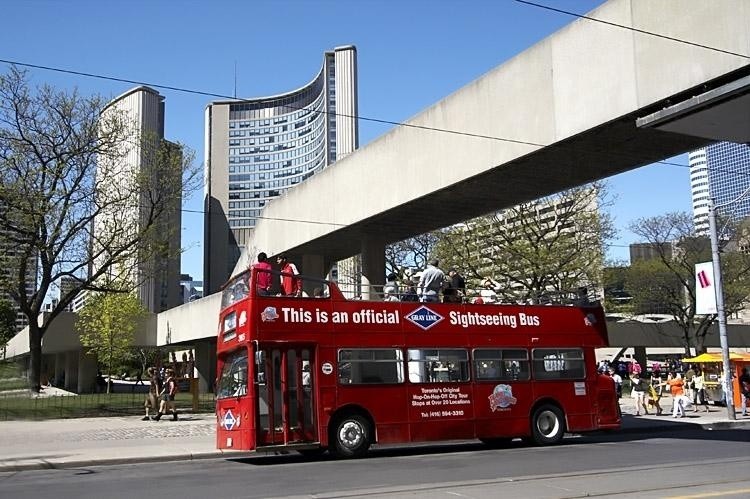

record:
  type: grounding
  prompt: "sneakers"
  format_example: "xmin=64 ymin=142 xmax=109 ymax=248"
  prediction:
xmin=142 ymin=413 xmax=177 ymax=421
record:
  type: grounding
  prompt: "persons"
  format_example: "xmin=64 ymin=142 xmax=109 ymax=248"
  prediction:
xmin=139 ymin=367 xmax=160 ymax=421
xmin=382 ymin=259 xmax=466 ymax=305
xmin=650 ymin=368 xmax=663 ymax=416
xmin=151 ymin=368 xmax=178 ymax=421
xmin=424 ymin=361 xmax=437 ymax=382
xmin=253 ymin=251 xmax=272 ymax=296
xmin=666 ymin=371 xmax=686 ymax=418
xmin=483 ymin=363 xmax=498 ymax=377
xmin=276 ymin=253 xmax=303 ymax=298
xmin=609 ymin=368 xmax=623 ymax=419
xmin=303 ymin=365 xmax=311 ymax=385
xmin=617 ymin=358 xmax=700 ymax=412
xmin=630 ymin=374 xmax=649 ymax=416
xmin=739 ymin=368 xmax=750 ymax=417
xmin=689 ymin=369 xmax=709 ymax=414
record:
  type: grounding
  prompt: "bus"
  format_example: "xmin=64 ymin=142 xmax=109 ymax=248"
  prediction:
xmin=213 ymin=267 xmax=621 ymax=459
